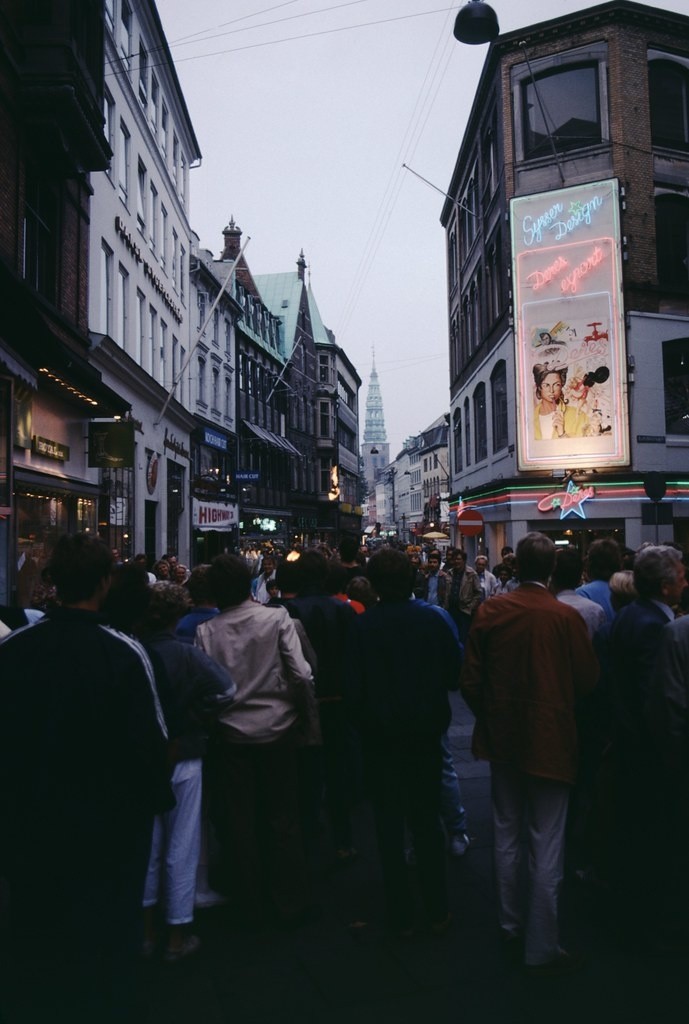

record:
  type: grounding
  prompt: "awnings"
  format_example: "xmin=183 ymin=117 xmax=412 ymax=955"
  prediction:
xmin=244 ymin=416 xmax=304 ymax=461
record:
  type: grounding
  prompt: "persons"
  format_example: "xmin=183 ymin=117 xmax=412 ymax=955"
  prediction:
xmin=0 ymin=522 xmax=689 ymax=1024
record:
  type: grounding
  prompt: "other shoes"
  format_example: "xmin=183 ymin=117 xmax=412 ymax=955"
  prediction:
xmin=450 ymin=833 xmax=469 ymax=856
xmin=140 ymin=940 xmax=154 ymax=955
xmin=167 ymin=936 xmax=200 ymax=958
xmin=529 ymin=950 xmax=570 ymax=973
xmin=431 ymin=913 xmax=453 ymax=928
xmin=195 ymin=888 xmax=226 ymax=908
